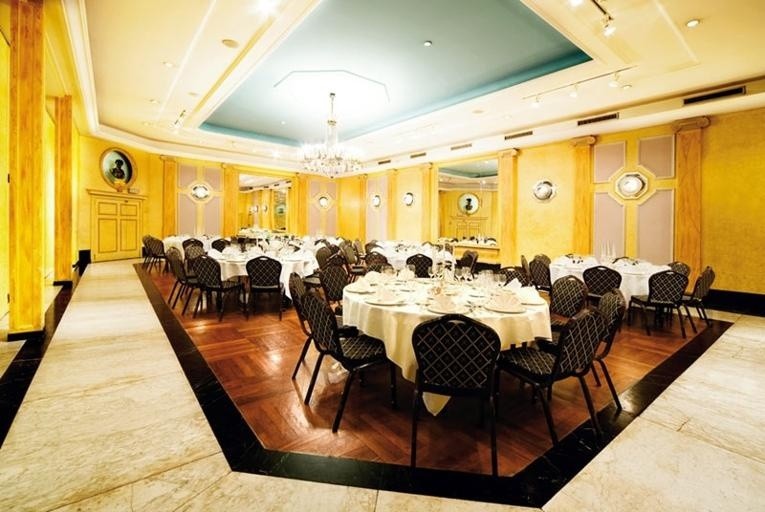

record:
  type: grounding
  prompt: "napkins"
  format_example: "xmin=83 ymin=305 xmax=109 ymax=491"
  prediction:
xmin=432 ymin=293 xmax=457 ymax=311
xmin=488 ymin=289 xmax=523 ymax=312
xmin=517 ymin=285 xmax=543 ymax=303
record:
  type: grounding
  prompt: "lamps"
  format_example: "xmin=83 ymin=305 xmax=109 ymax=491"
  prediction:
xmin=292 ymin=89 xmax=370 ymax=182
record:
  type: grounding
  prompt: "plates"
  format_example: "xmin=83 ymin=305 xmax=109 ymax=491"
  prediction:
xmin=211 ymin=252 xmax=303 ymax=262
xmin=346 ymin=279 xmax=546 ymax=316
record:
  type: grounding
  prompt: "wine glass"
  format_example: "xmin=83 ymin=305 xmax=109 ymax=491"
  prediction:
xmin=381 ymin=264 xmax=508 ymax=291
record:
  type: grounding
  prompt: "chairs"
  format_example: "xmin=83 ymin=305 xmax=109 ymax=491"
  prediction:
xmin=409 ymin=313 xmax=501 ymax=481
xmin=300 ymin=293 xmax=397 ymax=434
xmin=498 ymin=252 xmax=716 ymax=340
xmin=320 ymin=264 xmax=350 ymax=316
xmin=245 ymin=255 xmax=285 ymax=322
xmin=587 ymin=287 xmax=626 ymax=413
xmin=193 ymin=255 xmax=249 ymax=321
xmin=494 ymin=304 xmax=610 ymax=469
xmin=450 ymin=235 xmax=496 ymax=244
xmin=169 ymin=252 xmax=202 ymax=315
xmin=288 ymin=272 xmax=345 ymax=380
xmin=142 ymin=226 xmax=477 ymax=302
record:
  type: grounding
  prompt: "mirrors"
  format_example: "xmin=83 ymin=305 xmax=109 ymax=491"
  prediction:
xmin=438 ymin=158 xmax=498 ymax=248
